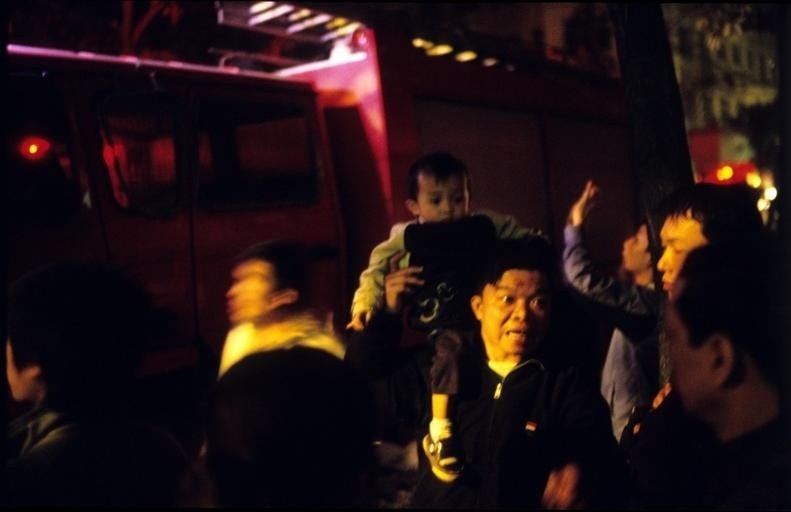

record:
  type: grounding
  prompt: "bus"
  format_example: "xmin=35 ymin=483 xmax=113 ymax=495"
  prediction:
xmin=4 ymin=43 xmax=353 ymax=362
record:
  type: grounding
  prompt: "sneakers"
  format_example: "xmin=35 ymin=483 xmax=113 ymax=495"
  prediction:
xmin=422 ymin=433 xmax=460 ymax=483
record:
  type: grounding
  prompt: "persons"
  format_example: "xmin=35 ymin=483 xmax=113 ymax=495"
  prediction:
xmin=3 ymin=258 xmax=179 ymax=510
xmin=205 ymin=152 xmax=791 ymax=510
